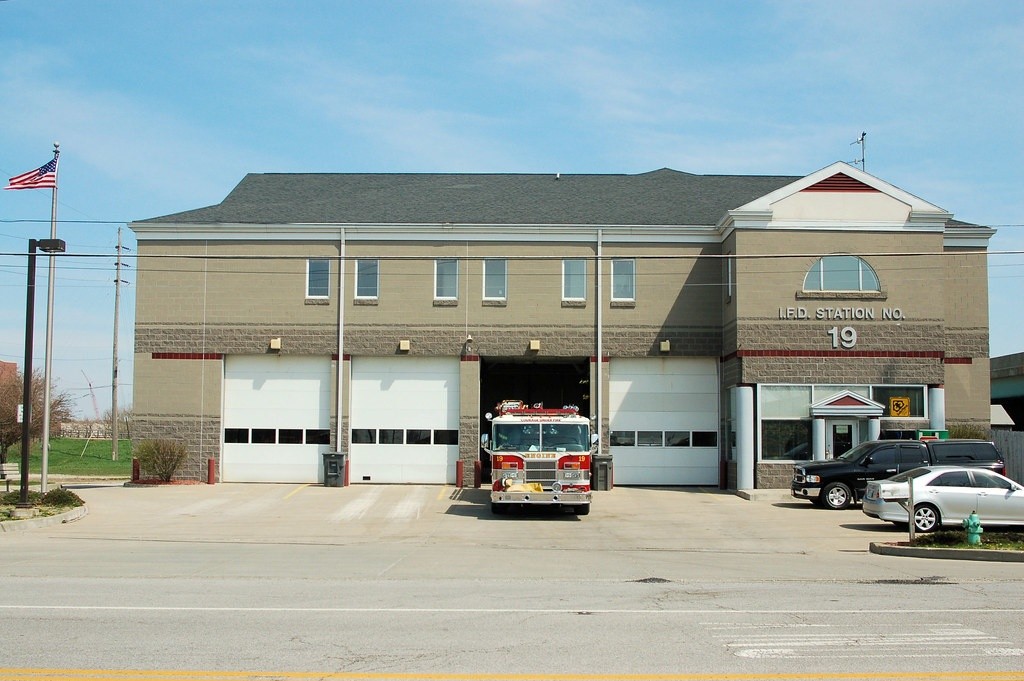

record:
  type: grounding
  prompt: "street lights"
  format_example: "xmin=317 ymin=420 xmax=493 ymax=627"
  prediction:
xmin=18 ymin=239 xmax=65 ymax=507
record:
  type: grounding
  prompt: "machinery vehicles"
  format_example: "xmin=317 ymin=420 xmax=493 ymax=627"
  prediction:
xmin=81 ymin=370 xmax=101 ymax=420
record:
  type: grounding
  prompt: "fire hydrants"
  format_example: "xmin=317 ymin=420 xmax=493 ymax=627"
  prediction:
xmin=962 ymin=511 xmax=983 ymax=545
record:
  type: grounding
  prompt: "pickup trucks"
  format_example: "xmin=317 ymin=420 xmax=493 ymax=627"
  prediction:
xmin=790 ymin=435 xmax=1006 ymax=510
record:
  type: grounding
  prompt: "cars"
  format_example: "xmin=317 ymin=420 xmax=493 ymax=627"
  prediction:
xmin=862 ymin=466 xmax=1024 ymax=532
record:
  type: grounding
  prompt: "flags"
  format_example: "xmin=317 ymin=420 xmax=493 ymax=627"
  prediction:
xmin=3 ymin=151 xmax=59 ymax=191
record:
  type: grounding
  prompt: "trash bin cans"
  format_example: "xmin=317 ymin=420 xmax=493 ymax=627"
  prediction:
xmin=322 ymin=451 xmax=347 ymax=487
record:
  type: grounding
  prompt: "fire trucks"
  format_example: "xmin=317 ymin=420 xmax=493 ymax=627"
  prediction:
xmin=481 ymin=400 xmax=600 ymax=514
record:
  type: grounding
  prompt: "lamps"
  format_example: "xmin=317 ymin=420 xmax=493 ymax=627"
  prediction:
xmin=465 ymin=335 xmax=473 ymax=352
